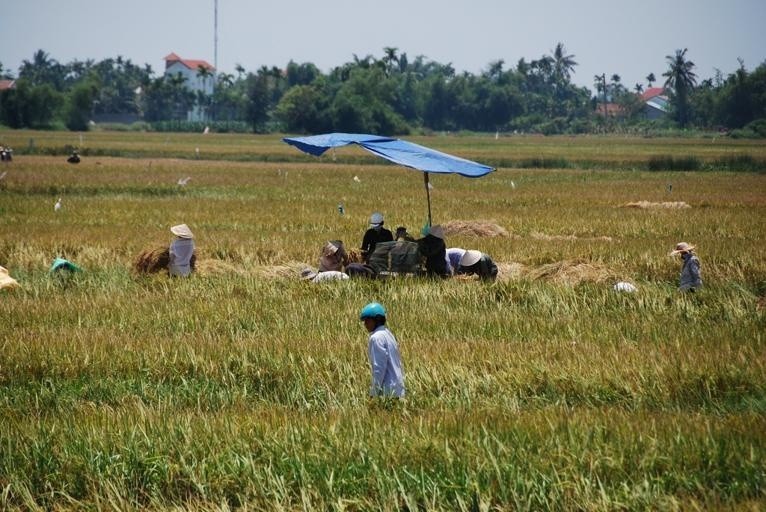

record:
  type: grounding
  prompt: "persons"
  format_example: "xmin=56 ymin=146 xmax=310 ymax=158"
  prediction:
xmin=67 ymin=151 xmax=81 ymax=163
xmin=168 ymin=223 xmax=194 ymax=279
xmin=360 ymin=213 xmax=498 ymax=289
xmin=359 ymin=303 xmax=405 ymax=411
xmin=318 ymin=240 xmax=348 ymax=274
xmin=667 ymin=242 xmax=702 ymax=292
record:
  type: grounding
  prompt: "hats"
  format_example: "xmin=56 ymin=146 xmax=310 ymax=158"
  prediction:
xmin=669 ymin=242 xmax=696 ymax=257
xmin=460 ymin=249 xmax=482 ymax=266
xmin=369 ymin=212 xmax=384 ymax=228
xmin=301 ymin=268 xmax=316 ymax=282
xmin=170 ymin=222 xmax=194 ymax=240
xmin=427 ymin=223 xmax=446 ymax=240
xmin=320 ymin=238 xmax=343 ymax=257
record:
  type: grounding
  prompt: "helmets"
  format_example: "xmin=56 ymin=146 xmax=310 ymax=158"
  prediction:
xmin=359 ymin=302 xmax=386 ymax=320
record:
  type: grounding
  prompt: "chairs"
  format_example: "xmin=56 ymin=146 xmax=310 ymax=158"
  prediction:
xmin=360 ymin=237 xmax=418 ymax=285
